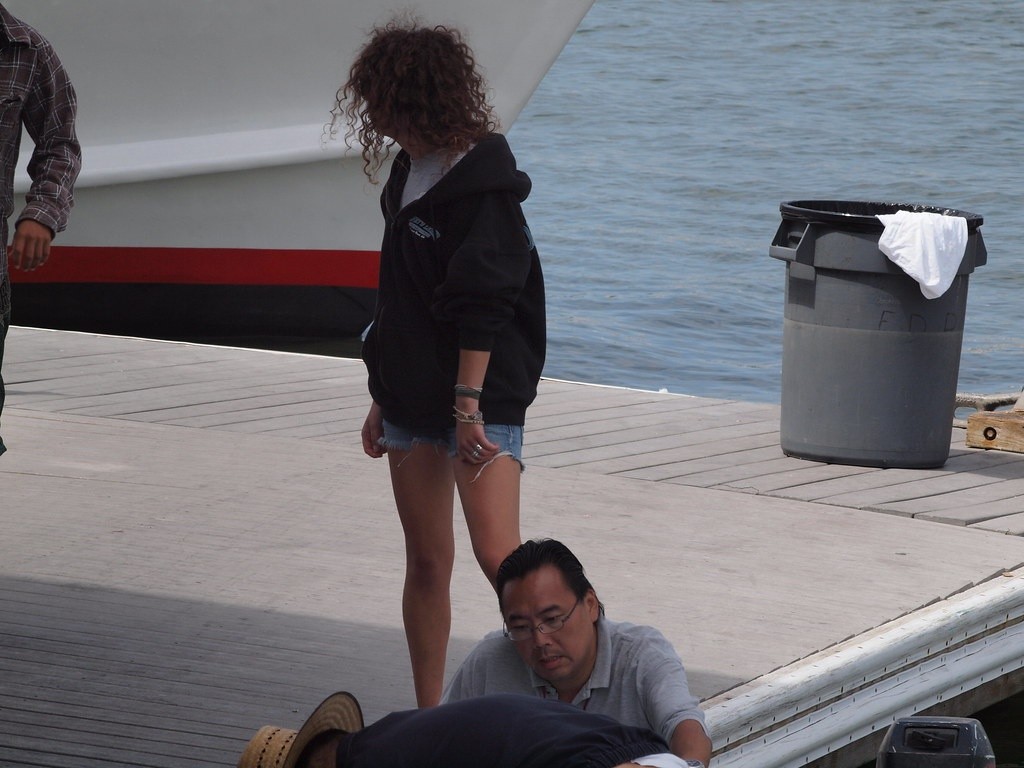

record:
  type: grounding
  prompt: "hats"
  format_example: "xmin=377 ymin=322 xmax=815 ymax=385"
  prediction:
xmin=237 ymin=691 xmax=365 ymax=768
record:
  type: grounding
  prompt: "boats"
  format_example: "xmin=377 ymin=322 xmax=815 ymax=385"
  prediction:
xmin=0 ymin=0 xmax=593 ymax=339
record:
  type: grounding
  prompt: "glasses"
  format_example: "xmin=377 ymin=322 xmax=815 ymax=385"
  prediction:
xmin=503 ymin=598 xmax=581 ymax=642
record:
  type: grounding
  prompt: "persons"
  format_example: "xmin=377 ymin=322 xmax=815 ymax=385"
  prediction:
xmin=330 ymin=22 xmax=546 ymax=709
xmin=242 ymin=541 xmax=712 ymax=766
xmin=1 ymin=8 xmax=83 ymax=454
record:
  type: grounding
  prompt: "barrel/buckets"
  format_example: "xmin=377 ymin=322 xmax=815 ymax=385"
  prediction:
xmin=769 ymin=200 xmax=989 ymax=470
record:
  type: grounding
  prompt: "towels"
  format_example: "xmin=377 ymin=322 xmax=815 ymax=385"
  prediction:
xmin=876 ymin=211 xmax=969 ymax=300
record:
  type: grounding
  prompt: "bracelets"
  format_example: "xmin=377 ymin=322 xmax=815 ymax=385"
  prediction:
xmin=453 ymin=385 xmax=484 ymax=424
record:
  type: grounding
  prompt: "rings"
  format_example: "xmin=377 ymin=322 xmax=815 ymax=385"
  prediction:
xmin=473 ymin=444 xmax=482 ymax=458
